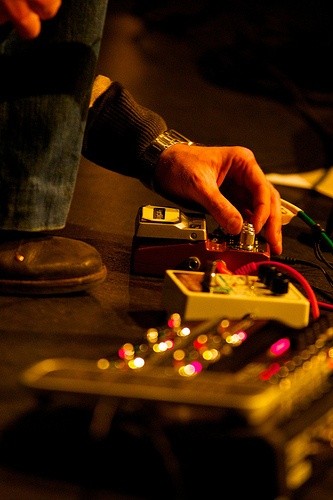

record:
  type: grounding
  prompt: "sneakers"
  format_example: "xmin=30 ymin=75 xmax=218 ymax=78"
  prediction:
xmin=0 ymin=233 xmax=107 ymax=294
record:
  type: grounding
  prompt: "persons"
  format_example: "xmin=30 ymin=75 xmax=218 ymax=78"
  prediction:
xmin=0 ymin=0 xmax=302 ymax=299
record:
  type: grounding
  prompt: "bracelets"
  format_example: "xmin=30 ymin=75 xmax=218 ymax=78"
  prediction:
xmin=138 ymin=129 xmax=195 ymax=190
